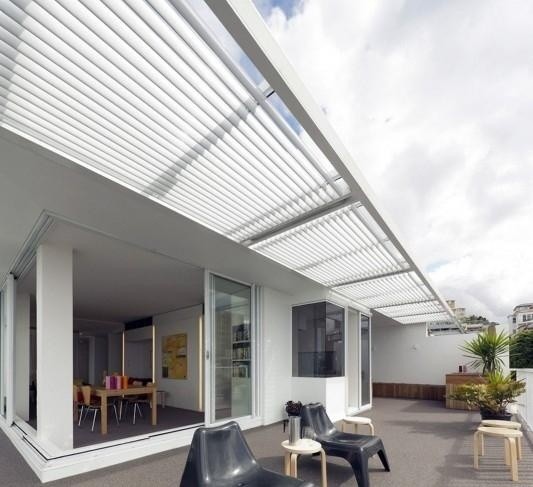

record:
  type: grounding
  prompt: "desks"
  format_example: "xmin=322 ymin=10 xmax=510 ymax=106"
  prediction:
xmin=94 ymin=385 xmax=158 ymax=435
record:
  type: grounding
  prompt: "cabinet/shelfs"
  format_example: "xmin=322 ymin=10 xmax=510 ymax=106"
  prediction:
xmin=231 ymin=323 xmax=250 ymax=379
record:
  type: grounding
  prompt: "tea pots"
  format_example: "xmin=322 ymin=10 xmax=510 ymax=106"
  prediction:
xmin=234 ymin=363 xmax=249 ymax=376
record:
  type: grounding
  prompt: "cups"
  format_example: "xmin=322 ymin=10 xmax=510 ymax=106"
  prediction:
xmin=301 ymin=426 xmax=317 ymax=448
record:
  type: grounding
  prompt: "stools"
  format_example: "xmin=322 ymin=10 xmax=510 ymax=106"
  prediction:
xmin=281 ymin=439 xmax=328 ymax=487
xmin=341 ymin=417 xmax=374 ymax=437
xmin=472 ymin=427 xmax=522 ymax=483
xmin=479 ymin=420 xmax=520 ymax=455
xmin=157 ymin=391 xmax=167 ymax=408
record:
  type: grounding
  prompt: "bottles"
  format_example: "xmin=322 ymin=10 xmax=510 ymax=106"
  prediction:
xmin=233 ymin=321 xmax=251 ymax=359
xmin=101 ymin=376 xmax=130 ymax=388
xmin=459 ymin=366 xmax=463 ymax=372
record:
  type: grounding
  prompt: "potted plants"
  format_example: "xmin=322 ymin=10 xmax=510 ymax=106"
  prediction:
xmin=445 ymin=362 xmax=527 ymax=427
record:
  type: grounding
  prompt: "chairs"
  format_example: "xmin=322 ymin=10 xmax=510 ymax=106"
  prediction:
xmin=301 ymin=402 xmax=390 ymax=487
xmin=179 ymin=421 xmax=320 ymax=487
xmin=73 ymin=377 xmax=152 ymax=432
